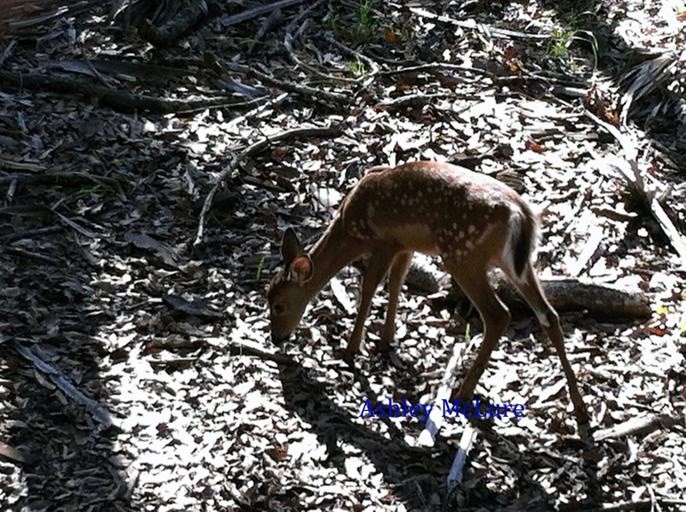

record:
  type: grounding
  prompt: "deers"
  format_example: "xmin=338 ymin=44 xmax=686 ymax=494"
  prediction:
xmin=266 ymin=159 xmax=592 ymax=426
